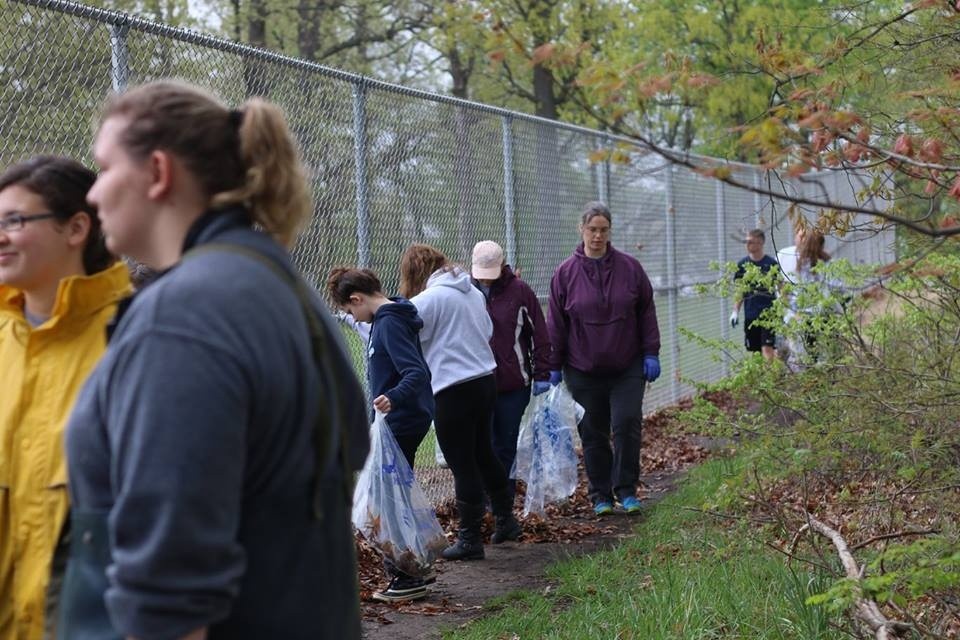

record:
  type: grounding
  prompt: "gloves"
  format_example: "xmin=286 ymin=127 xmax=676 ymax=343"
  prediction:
xmin=643 ymin=355 xmax=661 ymax=383
xmin=550 ymin=371 xmax=562 ymax=387
xmin=532 ymin=381 xmax=549 ymax=396
xmin=730 ymin=311 xmax=740 ymax=328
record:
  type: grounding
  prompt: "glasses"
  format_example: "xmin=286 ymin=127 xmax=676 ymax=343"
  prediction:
xmin=0 ymin=213 xmax=73 ymax=231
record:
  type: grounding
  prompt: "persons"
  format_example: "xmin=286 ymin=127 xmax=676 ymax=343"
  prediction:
xmin=731 ymin=225 xmax=856 ymax=372
xmin=339 ymin=242 xmax=522 ymax=559
xmin=328 ymin=266 xmax=437 ymax=603
xmin=45 ymin=81 xmax=365 ymax=640
xmin=545 ymin=202 xmax=661 ymax=516
xmin=0 ymin=155 xmax=136 ymax=640
xmin=471 ymin=240 xmax=550 ymax=512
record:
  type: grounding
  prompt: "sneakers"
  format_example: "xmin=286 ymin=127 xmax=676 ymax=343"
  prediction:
xmin=595 ymin=502 xmax=612 ymax=516
xmin=373 ymin=576 xmax=426 ymax=602
xmin=624 ymin=497 xmax=640 ymax=514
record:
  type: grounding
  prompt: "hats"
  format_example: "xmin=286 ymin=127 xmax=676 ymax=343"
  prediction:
xmin=471 ymin=240 xmax=503 ymax=279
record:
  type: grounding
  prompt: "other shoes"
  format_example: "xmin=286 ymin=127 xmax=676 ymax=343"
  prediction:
xmin=488 ymin=518 xmax=523 ymax=545
xmin=439 ymin=537 xmax=485 ymax=560
